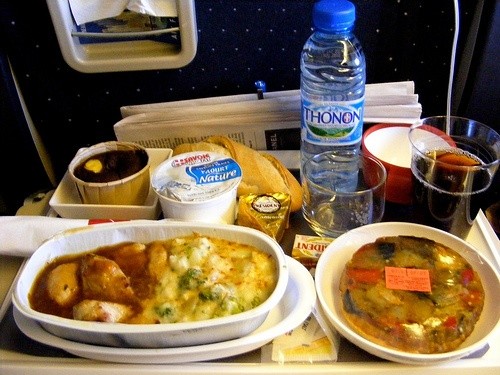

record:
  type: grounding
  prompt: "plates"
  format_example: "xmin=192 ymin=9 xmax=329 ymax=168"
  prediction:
xmin=14 ymin=254 xmax=316 ymax=364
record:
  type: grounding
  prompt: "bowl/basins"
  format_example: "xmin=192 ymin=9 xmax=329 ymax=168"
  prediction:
xmin=11 ymin=220 xmax=288 ymax=347
xmin=361 ymin=122 xmax=457 ymax=203
xmin=49 ymin=147 xmax=174 ymax=221
xmin=315 ymin=222 xmax=500 ymax=364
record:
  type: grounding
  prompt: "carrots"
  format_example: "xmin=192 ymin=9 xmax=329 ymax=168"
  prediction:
xmin=345 ymin=268 xmax=384 ymax=285
xmin=384 ymin=266 xmax=431 ymax=293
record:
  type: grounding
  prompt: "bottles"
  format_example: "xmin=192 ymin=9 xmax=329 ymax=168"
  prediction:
xmin=299 ymin=0 xmax=365 ymax=191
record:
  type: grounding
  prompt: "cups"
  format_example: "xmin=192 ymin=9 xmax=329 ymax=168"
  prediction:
xmin=301 ymin=149 xmax=387 ymax=238
xmin=150 ymin=150 xmax=243 ymax=225
xmin=409 ymin=116 xmax=500 ymax=243
xmin=69 ymin=141 xmax=150 ymax=205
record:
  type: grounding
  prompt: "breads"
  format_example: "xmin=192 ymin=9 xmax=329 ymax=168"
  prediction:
xmin=174 ymin=135 xmax=309 ymax=211
